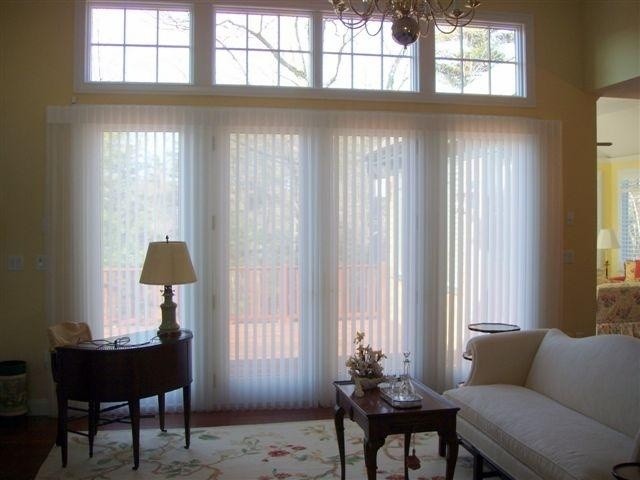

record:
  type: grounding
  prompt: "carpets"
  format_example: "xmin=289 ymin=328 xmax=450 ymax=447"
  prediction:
xmin=38 ymin=418 xmax=486 ymax=479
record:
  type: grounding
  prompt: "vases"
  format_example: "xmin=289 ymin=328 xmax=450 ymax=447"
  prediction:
xmin=351 ymin=376 xmax=387 ymax=391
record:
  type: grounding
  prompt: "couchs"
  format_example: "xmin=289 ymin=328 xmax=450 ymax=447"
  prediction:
xmin=443 ymin=328 xmax=639 ymax=479
xmin=597 ymin=284 xmax=640 ymax=335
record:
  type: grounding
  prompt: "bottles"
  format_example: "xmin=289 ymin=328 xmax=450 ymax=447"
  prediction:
xmin=397 ymin=351 xmax=415 ymax=401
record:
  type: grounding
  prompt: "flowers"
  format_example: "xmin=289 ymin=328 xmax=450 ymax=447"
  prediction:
xmin=344 ymin=330 xmax=392 ymax=398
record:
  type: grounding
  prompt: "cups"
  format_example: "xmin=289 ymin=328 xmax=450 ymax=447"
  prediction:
xmin=390 ymin=378 xmax=401 ymax=397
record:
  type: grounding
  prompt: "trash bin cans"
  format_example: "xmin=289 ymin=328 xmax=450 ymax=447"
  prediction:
xmin=0 ymin=361 xmax=28 ymax=418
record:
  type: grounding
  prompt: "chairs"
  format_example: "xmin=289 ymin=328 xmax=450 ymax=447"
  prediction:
xmin=46 ymin=322 xmax=133 ymax=460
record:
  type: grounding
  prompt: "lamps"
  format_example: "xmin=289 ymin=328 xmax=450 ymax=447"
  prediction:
xmin=327 ymin=0 xmax=482 ymax=46
xmin=596 ymin=230 xmax=622 ymax=281
xmin=138 ymin=238 xmax=199 ymax=338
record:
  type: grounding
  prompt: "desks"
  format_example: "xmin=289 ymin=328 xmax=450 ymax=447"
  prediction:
xmin=55 ymin=330 xmax=194 ymax=469
xmin=464 ymin=322 xmax=521 ymax=362
